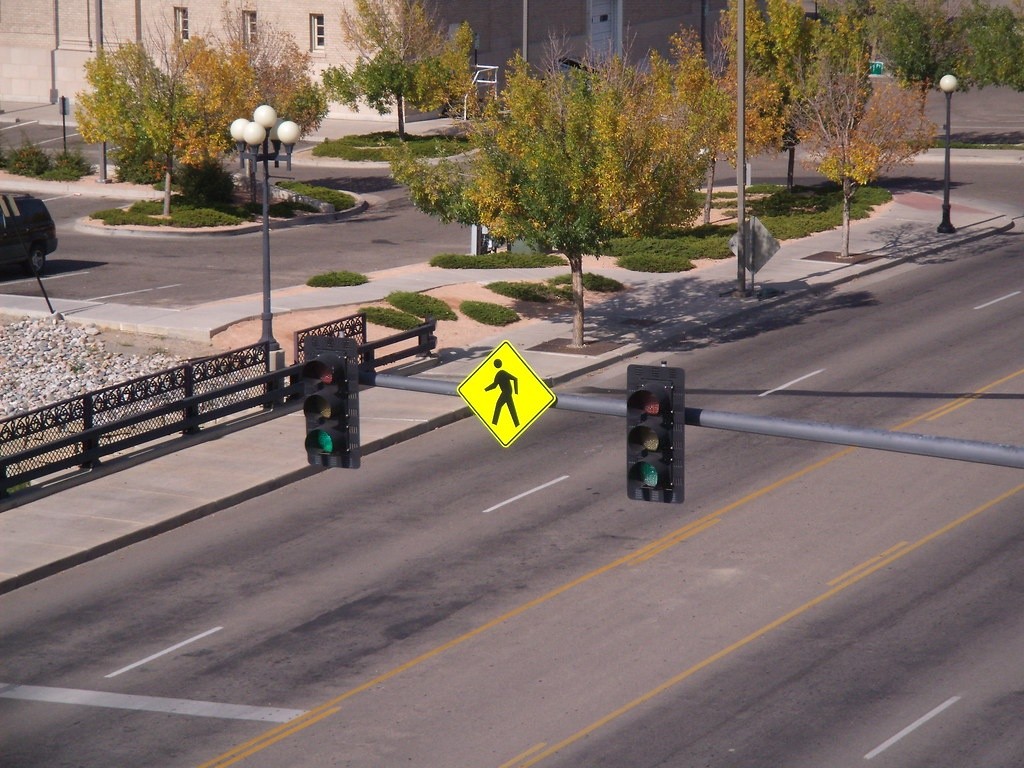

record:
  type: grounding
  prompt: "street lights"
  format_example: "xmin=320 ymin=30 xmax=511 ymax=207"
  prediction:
xmin=937 ymin=72 xmax=958 ymax=234
xmin=230 ymin=104 xmax=299 ymax=351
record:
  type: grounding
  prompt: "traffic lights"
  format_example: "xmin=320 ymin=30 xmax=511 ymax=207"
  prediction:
xmin=625 ymin=365 xmax=685 ymax=502
xmin=303 ymin=333 xmax=361 ymax=469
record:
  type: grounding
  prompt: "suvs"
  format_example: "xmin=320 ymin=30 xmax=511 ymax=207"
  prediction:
xmin=0 ymin=191 xmax=57 ymax=276
